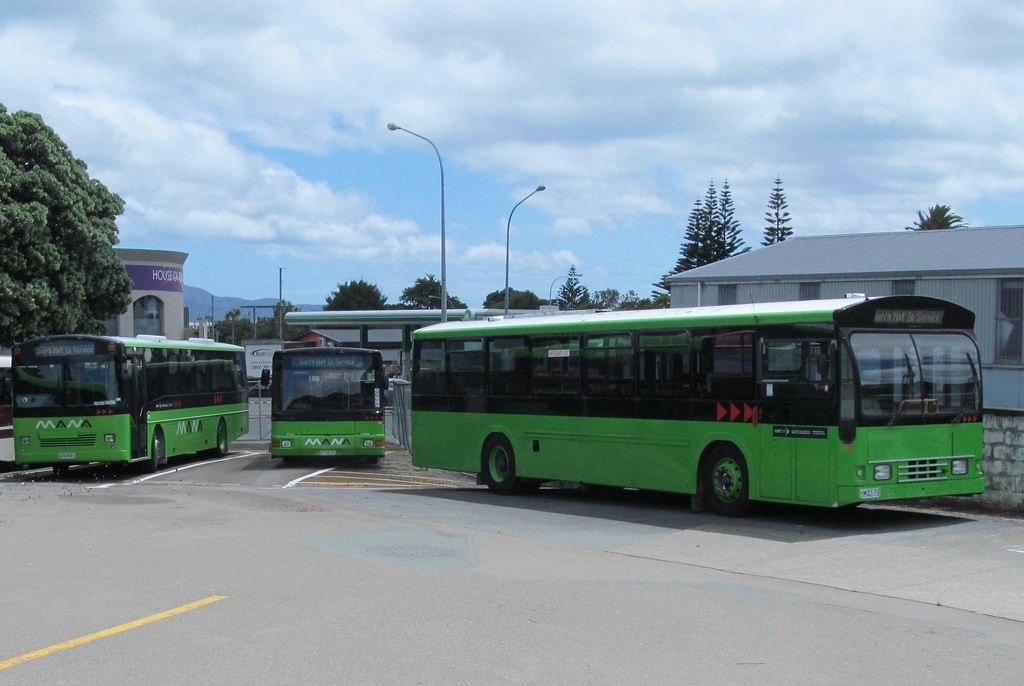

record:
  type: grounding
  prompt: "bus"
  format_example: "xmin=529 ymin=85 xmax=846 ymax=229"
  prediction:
xmin=412 ymin=291 xmax=985 ymax=521
xmin=259 ymin=347 xmax=389 ymax=465
xmin=11 ymin=335 xmax=249 ymax=478
xmin=0 ymin=346 xmax=107 ymax=468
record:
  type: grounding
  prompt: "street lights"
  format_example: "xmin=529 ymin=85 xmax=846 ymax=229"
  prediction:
xmin=504 ymin=185 xmax=547 ymax=312
xmin=387 ymin=123 xmax=448 ymax=373
xmin=550 ymin=274 xmax=583 ymax=305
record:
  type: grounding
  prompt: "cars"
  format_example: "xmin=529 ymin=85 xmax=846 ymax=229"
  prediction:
xmin=383 ymin=378 xmax=411 ymax=407
